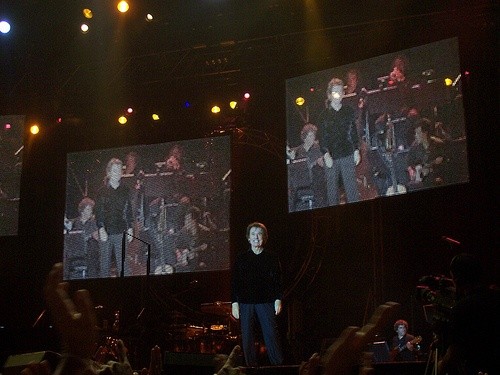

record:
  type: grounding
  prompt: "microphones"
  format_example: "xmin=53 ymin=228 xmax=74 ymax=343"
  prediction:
xmin=442 ymin=235 xmax=464 ymax=248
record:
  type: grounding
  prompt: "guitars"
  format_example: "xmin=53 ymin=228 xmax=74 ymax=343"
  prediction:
xmin=389 ymin=335 xmax=422 ymax=361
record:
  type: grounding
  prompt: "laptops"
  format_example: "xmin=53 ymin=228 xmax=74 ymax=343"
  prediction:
xmin=373 ymin=342 xmax=391 ymax=362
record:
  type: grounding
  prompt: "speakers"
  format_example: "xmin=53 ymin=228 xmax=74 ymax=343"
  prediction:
xmin=4 ymin=351 xmax=63 ymax=375
xmin=163 ymin=351 xmax=217 ymax=375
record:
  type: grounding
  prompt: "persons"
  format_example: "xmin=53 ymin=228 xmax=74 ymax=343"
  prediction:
xmin=18 ymin=222 xmax=499 ymax=375
xmin=62 ymin=138 xmax=230 ymax=276
xmin=284 ymin=54 xmax=456 ymax=207
